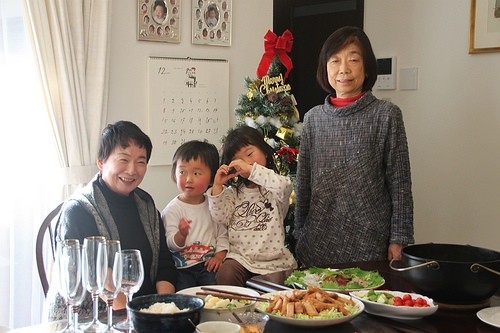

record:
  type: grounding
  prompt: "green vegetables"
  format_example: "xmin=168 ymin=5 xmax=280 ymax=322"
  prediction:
xmin=257 ymin=296 xmax=355 ymax=318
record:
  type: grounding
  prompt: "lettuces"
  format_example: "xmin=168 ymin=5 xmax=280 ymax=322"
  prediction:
xmin=285 ymin=266 xmax=384 ymax=289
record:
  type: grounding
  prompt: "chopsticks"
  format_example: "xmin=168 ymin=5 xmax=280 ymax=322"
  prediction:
xmin=196 ymin=287 xmax=273 ymax=302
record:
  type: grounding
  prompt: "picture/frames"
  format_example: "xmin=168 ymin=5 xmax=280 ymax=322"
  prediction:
xmin=469 ymin=0 xmax=500 ymax=54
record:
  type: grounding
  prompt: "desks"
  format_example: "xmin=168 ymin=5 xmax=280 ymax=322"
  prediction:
xmin=7 ymin=308 xmax=405 ymax=333
xmin=246 ymin=260 xmax=500 ymax=333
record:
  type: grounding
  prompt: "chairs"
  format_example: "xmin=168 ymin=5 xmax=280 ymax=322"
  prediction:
xmin=36 ymin=202 xmax=64 ymax=299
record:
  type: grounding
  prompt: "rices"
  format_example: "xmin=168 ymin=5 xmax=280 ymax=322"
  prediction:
xmin=139 ymin=301 xmax=190 ymax=313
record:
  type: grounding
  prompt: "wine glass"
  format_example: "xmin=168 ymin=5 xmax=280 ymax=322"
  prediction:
xmin=55 ymin=236 xmax=145 ymax=333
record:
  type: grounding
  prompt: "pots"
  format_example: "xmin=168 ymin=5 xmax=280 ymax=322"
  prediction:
xmin=389 ymin=243 xmax=500 ymax=305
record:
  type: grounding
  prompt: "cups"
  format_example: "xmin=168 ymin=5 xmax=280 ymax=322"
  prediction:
xmin=228 ymin=312 xmax=270 ymax=333
xmin=195 ymin=320 xmax=240 ymax=333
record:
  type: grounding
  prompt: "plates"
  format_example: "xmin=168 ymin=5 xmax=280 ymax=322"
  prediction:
xmin=476 ymin=306 xmax=500 ymax=329
xmin=349 ymin=291 xmax=439 ymax=320
xmin=255 ymin=290 xmax=365 ymax=329
xmin=291 ymin=269 xmax=386 ymax=291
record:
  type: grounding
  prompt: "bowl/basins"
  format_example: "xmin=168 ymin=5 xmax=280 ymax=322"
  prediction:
xmin=126 ymin=293 xmax=205 ymax=333
xmin=177 ymin=284 xmax=260 ymax=322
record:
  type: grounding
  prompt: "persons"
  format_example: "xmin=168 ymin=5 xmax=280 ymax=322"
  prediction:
xmin=294 ymin=26 xmax=416 ymax=269
xmin=206 ymin=125 xmax=297 ymax=291
xmin=142 ymin=0 xmax=177 ymax=37
xmin=196 ymin=0 xmax=229 ymax=40
xmin=40 ymin=121 xmax=178 ymax=321
xmin=160 ymin=139 xmax=228 ymax=286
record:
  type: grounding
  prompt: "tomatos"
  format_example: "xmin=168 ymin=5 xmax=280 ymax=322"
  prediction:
xmin=393 ymin=294 xmax=431 ymax=307
